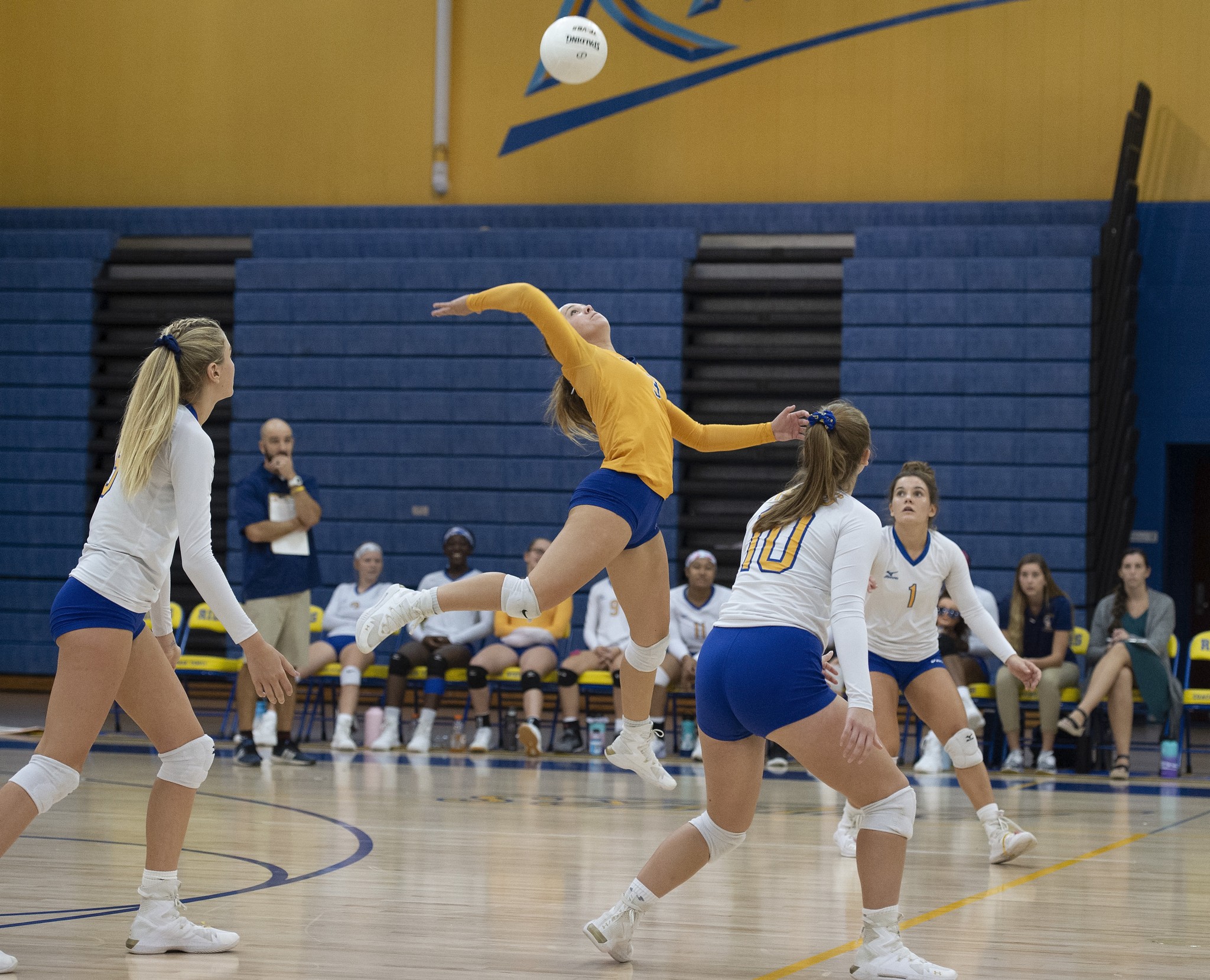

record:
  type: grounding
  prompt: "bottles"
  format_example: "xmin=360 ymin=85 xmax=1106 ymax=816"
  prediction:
xmin=363 ymin=702 xmax=383 ymax=750
xmin=448 ymin=715 xmax=467 ymax=752
xmin=251 ymin=702 xmax=266 ymax=729
xmin=503 ymin=708 xmax=518 ymax=751
xmin=679 ymin=714 xmax=695 ymax=758
xmin=407 ymin=714 xmax=418 ymax=743
xmin=1159 ymin=740 xmax=1179 ymax=778
xmin=588 ymin=729 xmax=602 ymax=755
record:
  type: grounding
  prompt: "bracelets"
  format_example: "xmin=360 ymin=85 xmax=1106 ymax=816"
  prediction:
xmin=291 ymin=485 xmax=306 ymax=495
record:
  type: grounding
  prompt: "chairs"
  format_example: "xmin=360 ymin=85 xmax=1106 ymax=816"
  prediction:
xmin=111 ymin=597 xmax=1210 ymax=775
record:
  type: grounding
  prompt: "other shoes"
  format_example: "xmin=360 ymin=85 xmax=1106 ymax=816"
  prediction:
xmin=690 ymin=737 xmax=704 ymax=762
xmin=1001 ymin=749 xmax=1026 ymax=773
xmin=405 ymin=726 xmax=430 ymax=753
xmin=519 ymin=721 xmax=543 ymax=756
xmin=470 ymin=726 xmax=496 ymax=751
xmin=331 ymin=751 xmax=488 ymax=790
xmin=914 ymin=731 xmax=950 ymax=773
xmin=273 ymin=731 xmax=316 ymax=765
xmin=371 ymin=720 xmax=400 ymax=749
xmin=555 ymin=721 xmax=585 ymax=753
xmin=332 ymin=722 xmax=356 ymax=750
xmin=234 ymin=732 xmax=262 ymax=766
xmin=961 ymin=695 xmax=985 ymax=731
xmin=650 ymin=738 xmax=666 ymax=758
xmin=1036 ymin=750 xmax=1057 ymax=775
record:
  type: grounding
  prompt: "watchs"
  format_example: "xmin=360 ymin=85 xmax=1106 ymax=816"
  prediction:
xmin=288 ymin=475 xmax=303 ymax=489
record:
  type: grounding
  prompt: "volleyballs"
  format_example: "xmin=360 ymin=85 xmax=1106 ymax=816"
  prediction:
xmin=539 ymin=15 xmax=608 ymax=85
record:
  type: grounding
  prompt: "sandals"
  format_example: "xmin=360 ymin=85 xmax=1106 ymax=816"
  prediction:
xmin=1109 ymin=755 xmax=1131 ymax=780
xmin=1057 ymin=707 xmax=1087 ymax=737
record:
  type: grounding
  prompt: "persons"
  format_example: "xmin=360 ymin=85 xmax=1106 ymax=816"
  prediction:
xmin=648 ymin=549 xmax=732 ymax=763
xmin=466 ymin=537 xmax=574 ymax=759
xmin=0 ymin=316 xmax=300 ymax=975
xmin=830 ymin=461 xmax=1036 ymax=865
xmin=231 ymin=417 xmax=322 ymax=767
xmin=553 ymin=577 xmax=631 ymax=755
xmin=367 ymin=525 xmax=494 ymax=753
xmin=1056 ymin=549 xmax=1184 ymax=782
xmin=233 ymin=541 xmax=394 ymax=750
xmin=767 ymin=622 xmax=847 ymax=767
xmin=580 ymin=399 xmax=959 ymax=980
xmin=912 ymin=548 xmax=999 ymax=773
xmin=993 ymin=553 xmax=1080 ymax=775
xmin=354 ymin=282 xmax=810 ymax=789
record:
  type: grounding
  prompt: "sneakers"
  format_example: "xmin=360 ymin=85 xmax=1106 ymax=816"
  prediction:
xmin=355 ymin=584 xmax=436 ymax=654
xmin=984 ymin=809 xmax=1037 ymax=864
xmin=603 ymin=721 xmax=679 ymax=792
xmin=850 ymin=911 xmax=958 ymax=980
xmin=0 ymin=950 xmax=18 ymax=972
xmin=833 ymin=799 xmax=862 ymax=857
xmin=583 ymin=887 xmax=653 ymax=963
xmin=125 ymin=880 xmax=240 ymax=955
